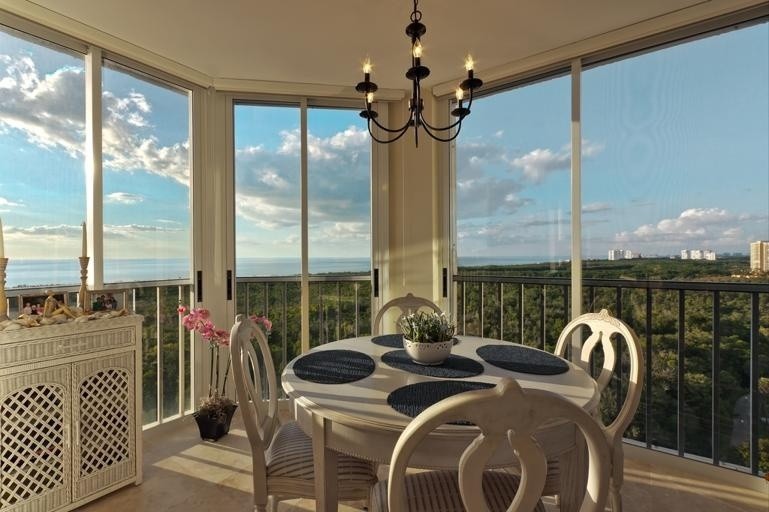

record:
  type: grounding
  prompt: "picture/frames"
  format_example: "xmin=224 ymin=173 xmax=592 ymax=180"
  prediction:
xmin=18 ymin=289 xmax=128 ymax=316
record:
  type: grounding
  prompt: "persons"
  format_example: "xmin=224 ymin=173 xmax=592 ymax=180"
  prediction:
xmin=30 ymin=304 xmax=40 ymax=316
xmin=92 ymin=294 xmax=117 ymax=311
xmin=41 ymin=289 xmax=77 ymax=320
xmin=24 ymin=301 xmax=32 ymax=316
xmin=35 ymin=303 xmax=44 ymax=316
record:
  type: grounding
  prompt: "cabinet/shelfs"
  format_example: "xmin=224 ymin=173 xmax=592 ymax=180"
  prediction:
xmin=0 ymin=307 xmax=148 ymax=512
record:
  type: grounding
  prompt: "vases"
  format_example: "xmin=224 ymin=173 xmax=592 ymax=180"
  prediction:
xmin=191 ymin=401 xmax=240 ymax=442
xmin=402 ymin=335 xmax=454 ymax=366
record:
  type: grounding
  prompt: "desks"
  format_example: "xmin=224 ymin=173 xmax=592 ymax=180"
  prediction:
xmin=280 ymin=332 xmax=603 ymax=510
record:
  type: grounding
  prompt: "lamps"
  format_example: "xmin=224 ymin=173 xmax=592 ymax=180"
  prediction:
xmin=356 ymin=0 xmax=484 ymax=151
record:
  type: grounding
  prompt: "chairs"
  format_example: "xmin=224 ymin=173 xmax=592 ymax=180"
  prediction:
xmin=230 ymin=314 xmax=380 ymax=510
xmin=499 ymin=307 xmax=645 ymax=510
xmin=373 ymin=373 xmax=611 ymax=510
xmin=371 ymin=292 xmax=448 ymax=337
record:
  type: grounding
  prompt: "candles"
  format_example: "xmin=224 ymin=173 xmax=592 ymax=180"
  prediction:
xmin=82 ymin=221 xmax=88 ymax=257
xmin=0 ymin=218 xmax=4 ymax=258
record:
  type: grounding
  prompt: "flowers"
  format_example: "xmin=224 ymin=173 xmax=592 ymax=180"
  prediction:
xmin=177 ymin=301 xmax=273 ymax=401
xmin=391 ymin=309 xmax=459 ymax=343
